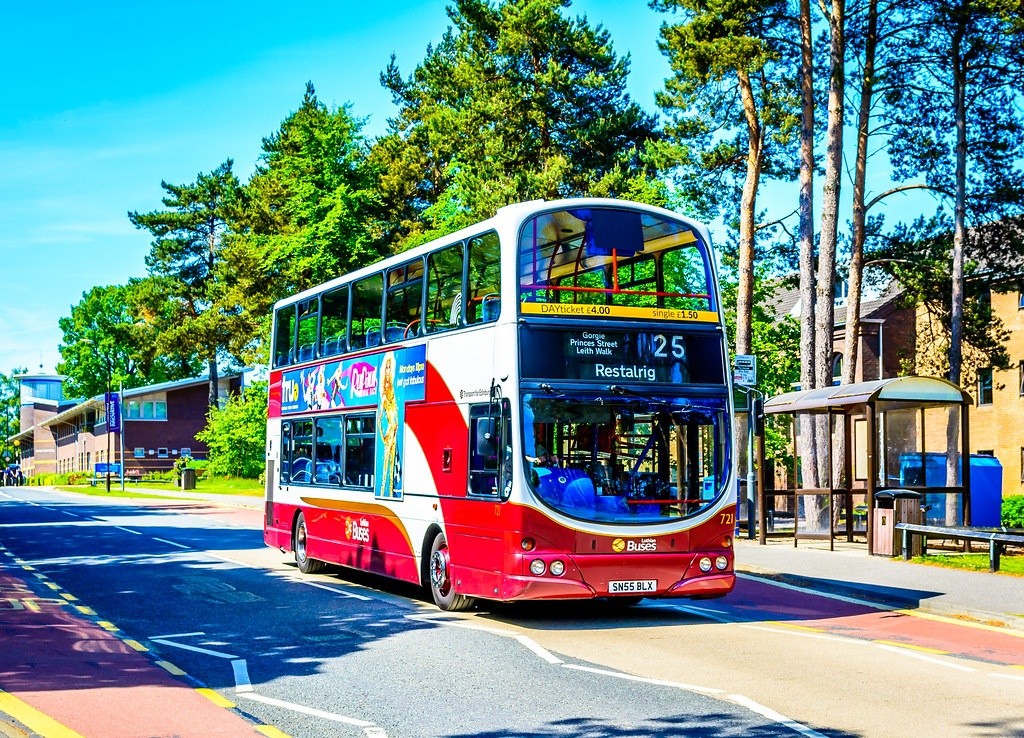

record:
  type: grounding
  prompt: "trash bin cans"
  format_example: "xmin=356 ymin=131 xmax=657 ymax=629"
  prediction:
xmin=873 ymin=490 xmax=922 ymax=558
xmin=181 ymin=468 xmax=195 ymax=490
xmin=703 ymin=476 xmax=717 ymax=499
xmin=898 ymin=451 xmax=1004 ymax=529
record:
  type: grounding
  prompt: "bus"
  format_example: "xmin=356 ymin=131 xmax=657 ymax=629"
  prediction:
xmin=262 ymin=197 xmax=767 ymax=612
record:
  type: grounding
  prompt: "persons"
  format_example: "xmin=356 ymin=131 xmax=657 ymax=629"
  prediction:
xmin=7 ymin=466 xmax=14 ymax=478
xmin=523 ymin=423 xmax=559 ymax=467
xmin=377 ymin=351 xmax=399 ymax=498
xmin=17 ymin=467 xmax=23 ymax=486
xmin=301 ymin=362 xmax=349 ymax=411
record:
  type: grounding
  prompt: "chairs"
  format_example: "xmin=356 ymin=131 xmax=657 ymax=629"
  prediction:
xmin=276 ymin=294 xmax=414 ymax=367
xmin=482 ymin=292 xmax=553 ymax=321
xmin=292 ymin=448 xmax=354 ymax=486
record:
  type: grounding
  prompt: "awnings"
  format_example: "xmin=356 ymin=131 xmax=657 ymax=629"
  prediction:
xmin=37 ymin=395 xmax=105 ymax=434
xmin=5 ymin=426 xmax=34 ymax=446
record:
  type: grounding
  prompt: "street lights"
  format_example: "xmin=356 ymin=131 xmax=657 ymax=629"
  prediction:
xmin=859 ymin=316 xmax=888 ymax=489
